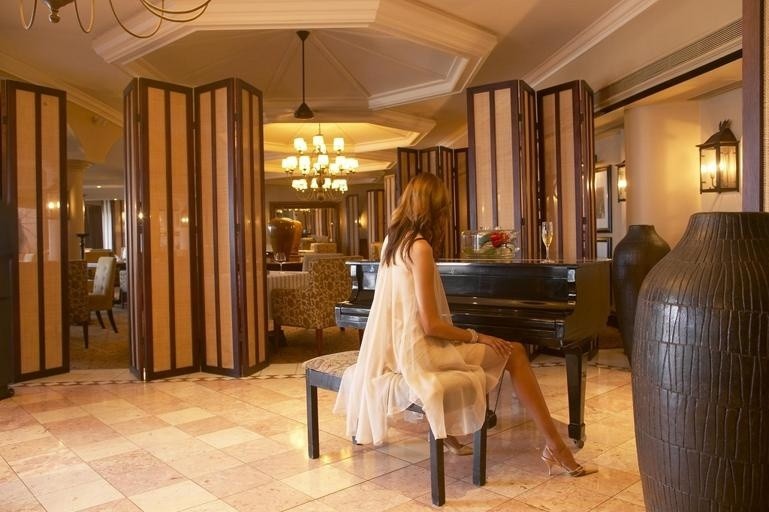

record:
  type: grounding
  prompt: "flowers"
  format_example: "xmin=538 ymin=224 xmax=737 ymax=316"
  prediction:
xmin=477 ymin=233 xmax=517 ymax=256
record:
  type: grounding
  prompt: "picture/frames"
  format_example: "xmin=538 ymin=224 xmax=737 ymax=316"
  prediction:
xmin=594 ymin=165 xmax=612 ymax=233
xmin=595 ymin=237 xmax=612 ymax=305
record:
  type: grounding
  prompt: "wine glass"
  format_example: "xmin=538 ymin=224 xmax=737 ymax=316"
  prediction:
xmin=275 ymin=252 xmax=286 ymax=271
xmin=539 ymin=221 xmax=556 ymax=265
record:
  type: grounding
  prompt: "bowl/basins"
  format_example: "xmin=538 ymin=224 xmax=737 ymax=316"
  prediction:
xmin=459 ymin=229 xmax=517 ymax=259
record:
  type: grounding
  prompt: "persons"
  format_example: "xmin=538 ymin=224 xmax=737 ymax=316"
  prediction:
xmin=333 ymin=171 xmax=599 ymax=476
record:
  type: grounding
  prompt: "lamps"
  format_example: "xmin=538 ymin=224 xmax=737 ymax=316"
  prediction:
xmin=615 ymin=160 xmax=626 ymax=203
xmin=294 ymin=31 xmax=313 ymax=119
xmin=282 ymin=123 xmax=359 ymax=201
xmin=696 ymin=120 xmax=740 ymax=194
xmin=20 ymin=0 xmax=211 ymax=38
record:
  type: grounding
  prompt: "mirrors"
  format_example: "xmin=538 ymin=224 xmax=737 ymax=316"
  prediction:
xmin=270 ymin=200 xmax=341 ymax=252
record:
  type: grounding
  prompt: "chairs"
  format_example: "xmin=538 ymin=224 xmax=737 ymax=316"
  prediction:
xmin=267 ymin=237 xmax=383 ymax=356
xmin=67 ymin=248 xmax=128 ymax=348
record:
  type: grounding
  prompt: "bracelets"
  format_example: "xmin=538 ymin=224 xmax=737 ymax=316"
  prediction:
xmin=466 ymin=328 xmax=478 ymax=344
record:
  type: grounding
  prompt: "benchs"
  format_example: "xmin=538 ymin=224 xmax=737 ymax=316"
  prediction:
xmin=301 ymin=350 xmax=489 ymax=506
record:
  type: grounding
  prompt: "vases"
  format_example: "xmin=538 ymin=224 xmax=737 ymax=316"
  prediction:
xmin=632 ymin=212 xmax=769 ymax=512
xmin=613 ymin=225 xmax=671 ymax=366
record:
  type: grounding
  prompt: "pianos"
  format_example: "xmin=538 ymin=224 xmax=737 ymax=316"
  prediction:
xmin=335 ymin=255 xmax=613 ymax=442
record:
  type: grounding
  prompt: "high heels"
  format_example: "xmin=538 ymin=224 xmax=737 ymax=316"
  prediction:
xmin=428 ymin=432 xmax=474 ymax=456
xmin=541 ymin=447 xmax=598 ymax=477
